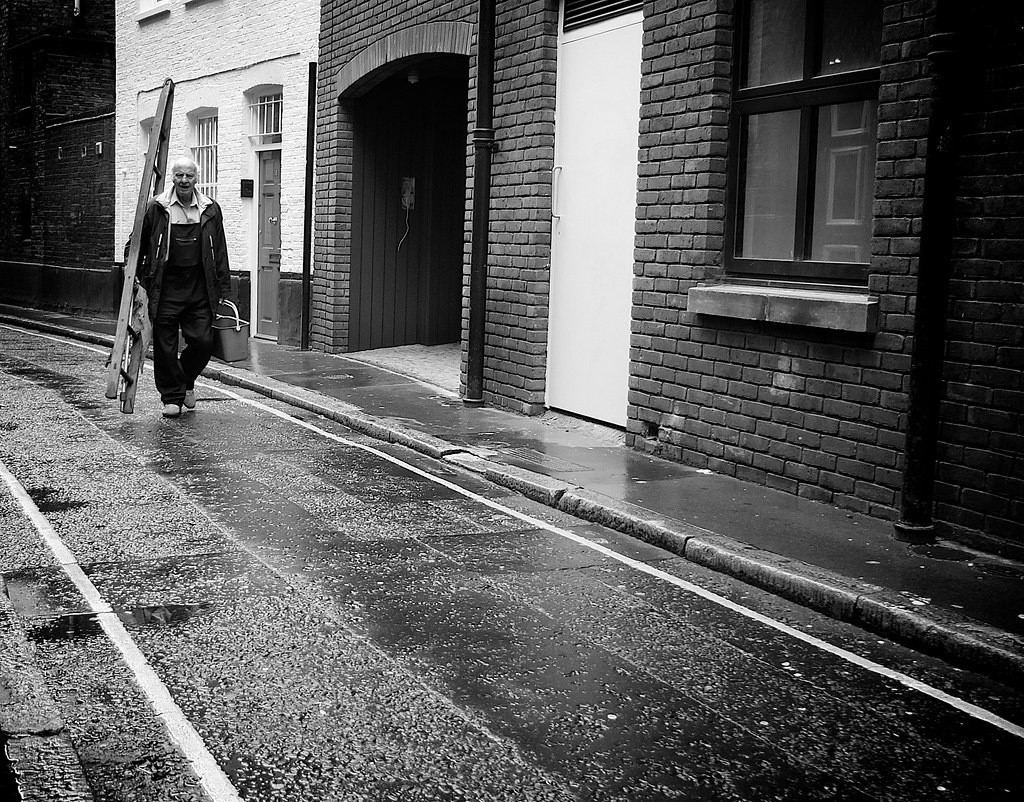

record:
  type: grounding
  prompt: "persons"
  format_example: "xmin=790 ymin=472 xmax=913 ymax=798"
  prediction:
xmin=124 ymin=158 xmax=232 ymax=417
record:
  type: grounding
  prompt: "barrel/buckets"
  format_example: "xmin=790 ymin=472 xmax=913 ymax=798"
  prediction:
xmin=203 ymin=300 xmax=251 ymax=364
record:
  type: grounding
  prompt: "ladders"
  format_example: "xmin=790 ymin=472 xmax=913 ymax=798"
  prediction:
xmin=104 ymin=77 xmax=175 ymax=414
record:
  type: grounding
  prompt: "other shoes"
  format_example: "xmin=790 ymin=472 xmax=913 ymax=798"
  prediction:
xmin=162 ymin=403 xmax=181 ymax=417
xmin=183 ymin=389 xmax=196 ymax=408
